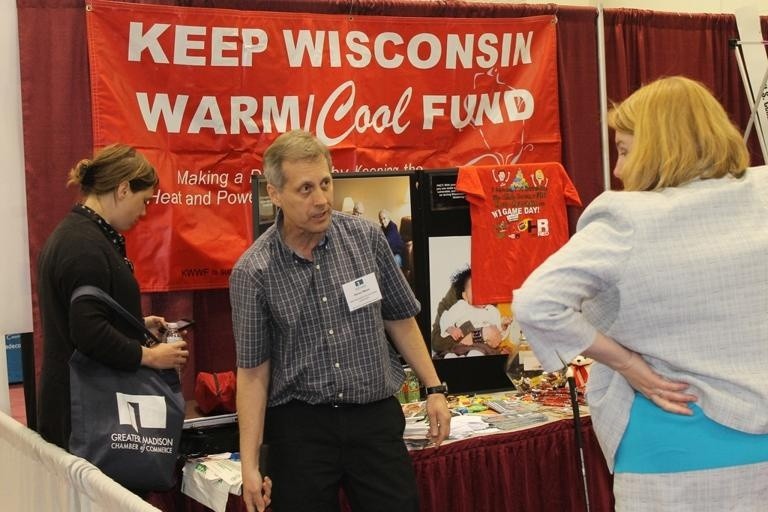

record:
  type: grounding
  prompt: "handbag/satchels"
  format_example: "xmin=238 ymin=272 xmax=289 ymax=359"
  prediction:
xmin=66 ymin=283 xmax=187 ymax=489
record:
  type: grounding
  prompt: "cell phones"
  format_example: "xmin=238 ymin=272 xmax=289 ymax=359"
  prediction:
xmin=159 ymin=317 xmax=195 ymax=334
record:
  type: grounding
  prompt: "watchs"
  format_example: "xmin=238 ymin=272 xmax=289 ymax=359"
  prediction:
xmin=419 ymin=381 xmax=449 ymax=398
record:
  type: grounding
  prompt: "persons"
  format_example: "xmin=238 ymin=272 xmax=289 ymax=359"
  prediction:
xmin=511 ymin=76 xmax=768 ymax=512
xmin=36 ymin=142 xmax=190 ymax=501
xmin=352 ymin=201 xmax=363 ymax=218
xmin=229 ymin=130 xmax=452 ymax=512
xmin=432 ymin=267 xmax=506 ymax=360
xmin=379 ymin=209 xmax=407 ymax=270
xmin=439 ymin=270 xmax=512 ymax=358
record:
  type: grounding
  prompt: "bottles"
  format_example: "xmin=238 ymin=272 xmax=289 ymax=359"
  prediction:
xmin=163 ymin=324 xmax=182 ymax=384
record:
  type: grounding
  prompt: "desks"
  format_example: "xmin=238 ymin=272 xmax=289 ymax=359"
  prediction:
xmin=174 ymin=390 xmax=614 ymax=509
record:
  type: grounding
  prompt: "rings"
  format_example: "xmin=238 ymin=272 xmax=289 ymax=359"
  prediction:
xmin=657 ymin=390 xmax=664 ymax=398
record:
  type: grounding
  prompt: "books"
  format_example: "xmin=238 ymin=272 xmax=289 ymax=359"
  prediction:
xmin=483 ymin=398 xmax=549 ymax=432
